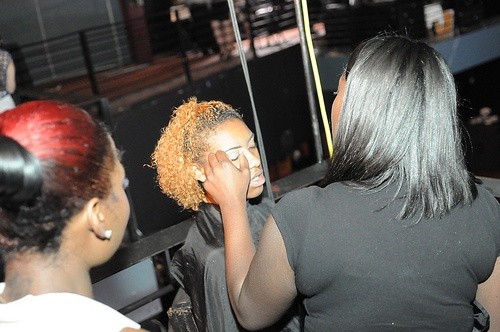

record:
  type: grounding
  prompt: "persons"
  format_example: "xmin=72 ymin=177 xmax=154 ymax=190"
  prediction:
xmin=0 ymin=50 xmax=16 ymax=115
xmin=0 ymin=100 xmax=154 ymax=331
xmin=152 ymin=97 xmax=305 ymax=332
xmin=194 ymin=33 xmax=500 ymax=332
